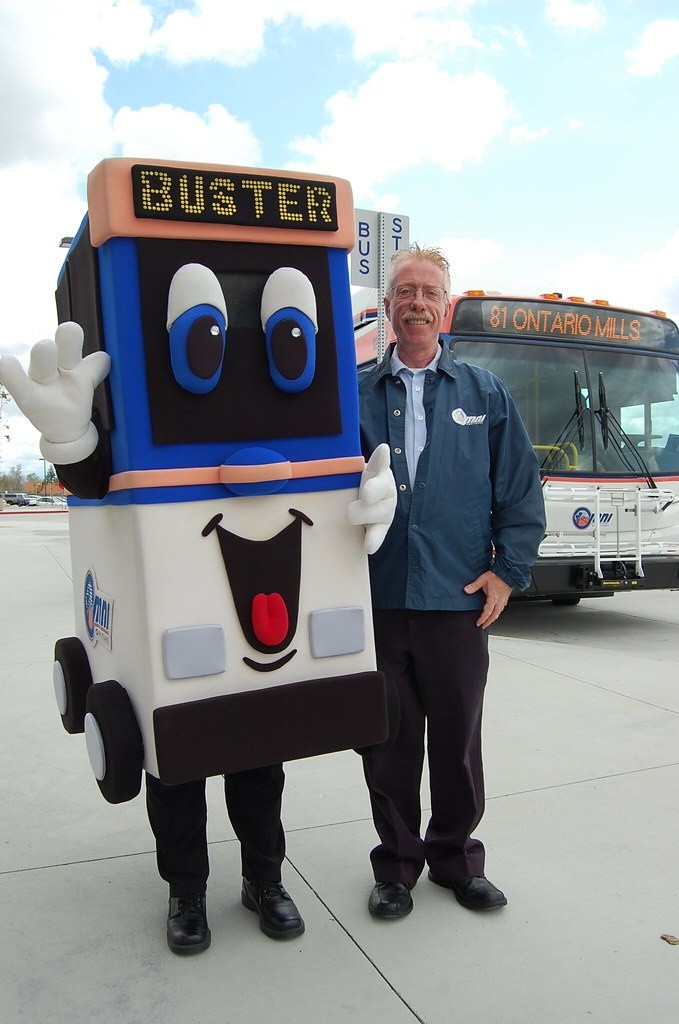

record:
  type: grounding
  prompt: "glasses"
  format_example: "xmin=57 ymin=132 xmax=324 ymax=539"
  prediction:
xmin=386 ymin=283 xmax=450 ymax=303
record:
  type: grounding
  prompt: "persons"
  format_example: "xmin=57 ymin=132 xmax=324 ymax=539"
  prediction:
xmin=362 ymin=241 xmax=547 ymax=917
xmin=0 ymin=157 xmax=398 ymax=954
xmin=550 ymin=394 xmax=608 ymax=451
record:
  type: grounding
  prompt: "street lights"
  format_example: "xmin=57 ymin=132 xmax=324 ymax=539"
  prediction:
xmin=39 ymin=459 xmax=46 ymax=496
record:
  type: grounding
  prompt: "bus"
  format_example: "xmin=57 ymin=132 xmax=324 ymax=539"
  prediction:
xmin=352 ymin=291 xmax=679 ymax=606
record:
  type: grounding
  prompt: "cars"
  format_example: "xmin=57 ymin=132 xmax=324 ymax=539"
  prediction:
xmin=0 ymin=493 xmax=67 ymax=506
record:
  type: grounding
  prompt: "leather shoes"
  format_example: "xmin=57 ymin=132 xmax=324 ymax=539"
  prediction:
xmin=241 ymin=877 xmax=305 ymax=938
xmin=427 ymin=866 xmax=507 ymax=910
xmin=368 ymin=879 xmax=414 ymax=918
xmin=167 ymin=889 xmax=211 ymax=954
